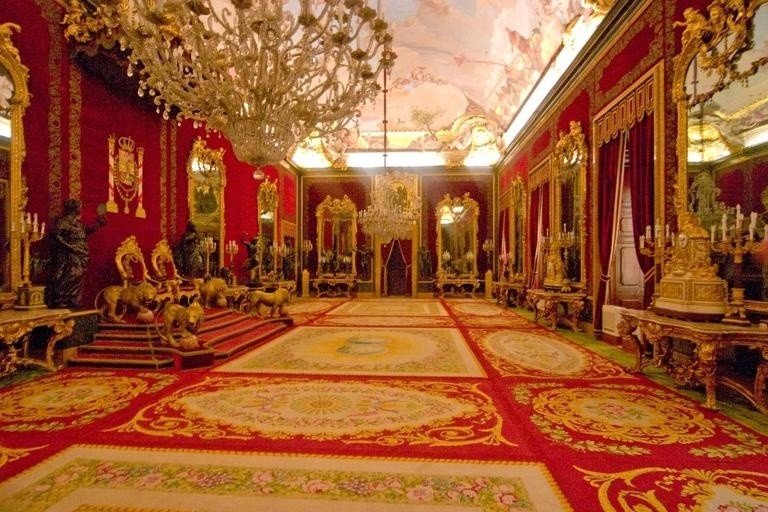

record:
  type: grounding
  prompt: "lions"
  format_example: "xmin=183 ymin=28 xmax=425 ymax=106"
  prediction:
xmin=196 ymin=278 xmax=229 ymax=309
xmin=94 ymin=282 xmax=157 ymax=324
xmin=154 ymin=301 xmax=205 ymax=347
xmin=246 ymin=288 xmax=291 ymax=319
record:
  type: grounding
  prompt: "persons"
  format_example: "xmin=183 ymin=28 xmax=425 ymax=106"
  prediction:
xmin=180 ymin=223 xmax=203 ymax=277
xmin=241 ymin=231 xmax=259 ymax=283
xmin=55 ymin=198 xmax=107 ymax=310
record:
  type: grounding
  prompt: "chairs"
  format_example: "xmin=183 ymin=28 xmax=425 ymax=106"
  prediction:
xmin=92 ymin=234 xmax=203 ymax=324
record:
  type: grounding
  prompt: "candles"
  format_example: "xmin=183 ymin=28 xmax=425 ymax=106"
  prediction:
xmin=545 ymin=223 xmax=575 ymax=241
xmin=638 ymin=223 xmax=675 ymax=249
xmin=710 ymin=203 xmax=758 ymax=243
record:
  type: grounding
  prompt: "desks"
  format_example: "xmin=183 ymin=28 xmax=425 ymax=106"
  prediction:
xmin=616 ymin=310 xmax=768 ymax=418
xmin=0 ymin=308 xmax=72 ymax=377
xmin=525 ymin=290 xmax=587 ymax=334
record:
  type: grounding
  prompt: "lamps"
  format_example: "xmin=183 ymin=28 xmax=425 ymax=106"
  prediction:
xmin=63 ymin=0 xmax=399 ymax=182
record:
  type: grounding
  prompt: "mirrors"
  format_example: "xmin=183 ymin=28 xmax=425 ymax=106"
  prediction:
xmin=256 ymin=182 xmax=279 ymax=280
xmin=435 ymin=192 xmax=480 ymax=280
xmin=184 ymin=140 xmax=228 ymax=272
xmin=315 ymin=193 xmax=360 ymax=278
